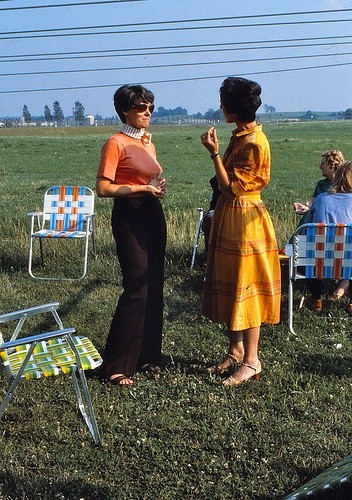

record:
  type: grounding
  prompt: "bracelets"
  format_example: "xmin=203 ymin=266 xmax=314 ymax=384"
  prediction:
xmin=210 ymin=152 xmax=220 ymax=159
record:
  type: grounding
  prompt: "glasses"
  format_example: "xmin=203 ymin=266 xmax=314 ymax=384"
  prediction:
xmin=129 ymin=103 xmax=155 ymax=113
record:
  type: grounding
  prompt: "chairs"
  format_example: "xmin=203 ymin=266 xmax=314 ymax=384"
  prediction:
xmin=26 ymin=185 xmax=98 ymax=281
xmin=287 ymin=223 xmax=352 ymax=349
xmin=0 ymin=302 xmax=105 ymax=447
xmin=190 ymin=208 xmax=207 ymax=270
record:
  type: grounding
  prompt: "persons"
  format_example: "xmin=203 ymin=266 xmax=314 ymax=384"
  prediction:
xmin=96 ymin=84 xmax=168 ymax=387
xmin=199 ymin=76 xmax=352 ymax=387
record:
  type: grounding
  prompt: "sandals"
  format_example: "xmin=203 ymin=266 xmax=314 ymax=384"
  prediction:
xmin=136 ymin=362 xmax=161 ymax=374
xmin=105 ymin=372 xmax=135 ymax=386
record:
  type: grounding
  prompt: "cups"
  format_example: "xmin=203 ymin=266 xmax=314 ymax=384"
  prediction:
xmin=295 ymin=199 xmax=303 ymax=211
xmin=151 ymin=179 xmax=160 ymax=188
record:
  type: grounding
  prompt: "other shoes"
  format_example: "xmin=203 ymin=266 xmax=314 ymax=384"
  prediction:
xmin=327 ymin=292 xmax=340 ymax=303
xmin=307 ymin=295 xmax=322 ymax=311
xmin=346 ymin=302 xmax=352 ymax=314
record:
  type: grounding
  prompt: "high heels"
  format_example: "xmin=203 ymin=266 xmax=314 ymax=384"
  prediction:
xmin=206 ymin=351 xmax=243 ymax=377
xmin=220 ymin=360 xmax=262 ymax=386
